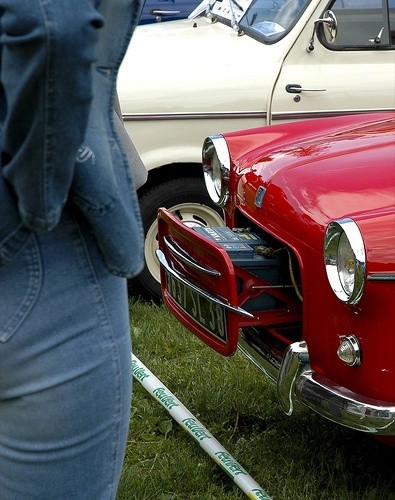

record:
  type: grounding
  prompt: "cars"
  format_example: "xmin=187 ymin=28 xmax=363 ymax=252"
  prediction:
xmin=156 ymin=111 xmax=395 ymax=447
xmin=115 ymin=0 xmax=395 ymax=308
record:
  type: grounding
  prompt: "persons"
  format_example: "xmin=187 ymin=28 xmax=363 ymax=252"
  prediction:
xmin=1 ymin=0 xmax=152 ymax=500
